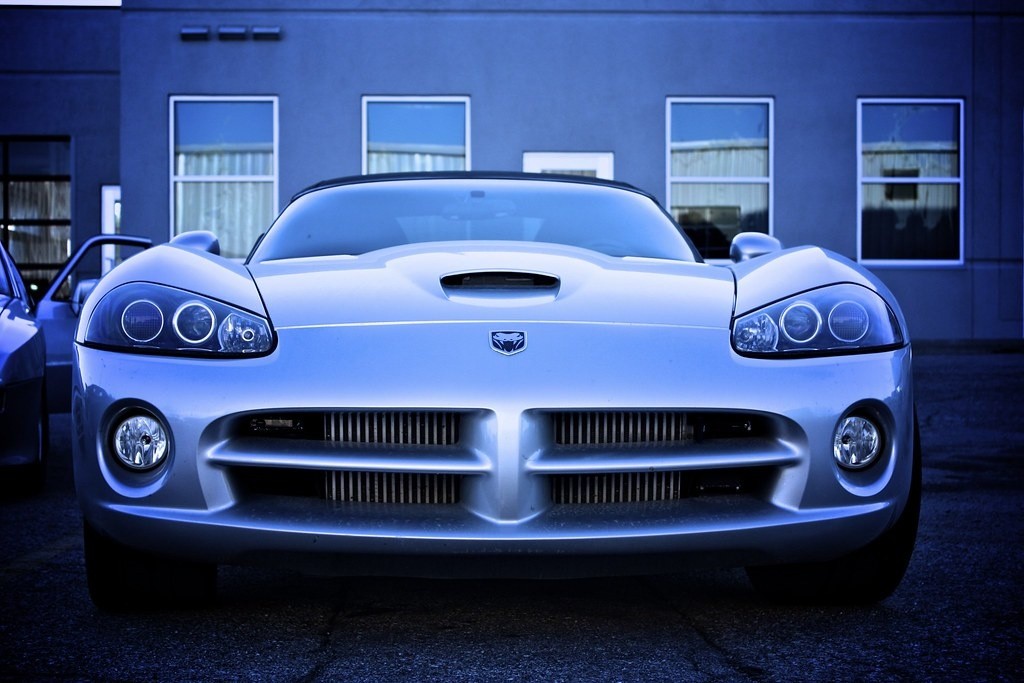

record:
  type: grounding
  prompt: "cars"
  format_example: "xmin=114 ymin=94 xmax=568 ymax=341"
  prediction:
xmin=0 ymin=232 xmax=161 ymax=471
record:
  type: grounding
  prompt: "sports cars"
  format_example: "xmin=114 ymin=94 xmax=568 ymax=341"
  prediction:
xmin=64 ymin=168 xmax=921 ymax=601
